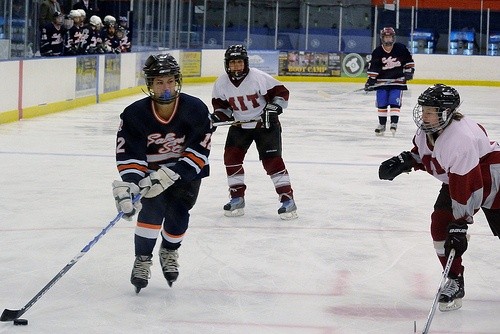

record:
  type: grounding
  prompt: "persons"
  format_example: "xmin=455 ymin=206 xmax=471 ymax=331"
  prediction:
xmin=32 ymin=0 xmax=132 ymax=57
xmin=379 ymin=83 xmax=500 ymax=311
xmin=112 ymin=54 xmax=217 ymax=294
xmin=364 ymin=27 xmax=415 ymax=135
xmin=287 ymin=51 xmax=313 ymax=68
xmin=211 ymin=44 xmax=298 ymax=219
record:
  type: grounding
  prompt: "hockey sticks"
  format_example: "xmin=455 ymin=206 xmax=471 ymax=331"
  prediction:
xmin=413 ymin=234 xmax=470 ymax=334
xmin=0 ymin=180 xmax=159 ymax=322
xmin=352 ymin=77 xmax=405 ymax=93
xmin=212 ymin=119 xmax=261 ymax=127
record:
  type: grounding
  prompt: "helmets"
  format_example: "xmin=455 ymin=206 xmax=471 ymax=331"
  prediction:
xmin=68 ymin=9 xmax=82 ymax=26
xmin=52 ymin=11 xmax=64 ymax=26
xmin=411 ymin=83 xmax=462 ymax=135
xmin=90 ymin=14 xmax=105 ymax=30
xmin=76 ymin=8 xmax=87 ymax=22
xmin=223 ymin=43 xmax=250 ymax=81
xmin=139 ymin=54 xmax=183 ymax=105
xmin=103 ymin=14 xmax=117 ymax=27
xmin=379 ymin=26 xmax=397 ymax=48
xmin=118 ymin=15 xmax=127 ymax=27
xmin=61 ymin=14 xmax=74 ymax=29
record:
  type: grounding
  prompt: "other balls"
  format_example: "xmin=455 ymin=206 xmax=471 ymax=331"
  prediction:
xmin=14 ymin=319 xmax=28 ymax=326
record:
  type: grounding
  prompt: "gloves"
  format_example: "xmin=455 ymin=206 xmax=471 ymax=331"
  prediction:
xmin=110 ymin=179 xmax=143 ymax=222
xmin=378 ymin=149 xmax=413 ymax=181
xmin=210 ymin=113 xmax=220 ymax=130
xmin=444 ymin=222 xmax=470 ymax=259
xmin=402 ymin=67 xmax=416 ymax=79
xmin=137 ymin=166 xmax=182 ymax=199
xmin=365 ymin=77 xmax=377 ymax=93
xmin=261 ymin=103 xmax=284 ymax=132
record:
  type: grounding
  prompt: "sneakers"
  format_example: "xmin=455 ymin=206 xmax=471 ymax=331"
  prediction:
xmin=130 ymin=253 xmax=153 ymax=293
xmin=373 ymin=123 xmax=387 ymax=137
xmin=391 ymin=123 xmax=400 ymax=136
xmin=223 ymin=195 xmax=246 ymax=217
xmin=157 ymin=247 xmax=180 ymax=288
xmin=276 ymin=198 xmax=298 ymax=221
xmin=437 ymin=265 xmax=466 ymax=312
